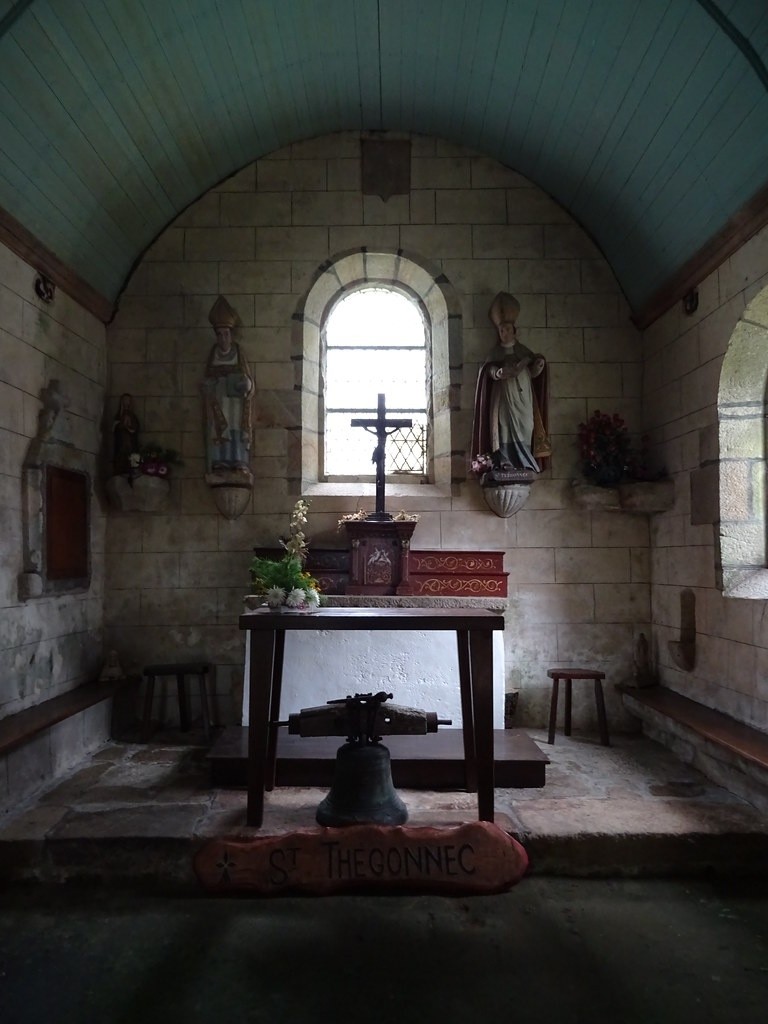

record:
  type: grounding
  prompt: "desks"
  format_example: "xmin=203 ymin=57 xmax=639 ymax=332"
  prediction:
xmin=238 ymin=605 xmax=504 ymax=828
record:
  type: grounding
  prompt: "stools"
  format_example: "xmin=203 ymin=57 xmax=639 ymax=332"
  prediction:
xmin=141 ymin=663 xmax=211 ymax=741
xmin=546 ymin=668 xmax=610 ymax=747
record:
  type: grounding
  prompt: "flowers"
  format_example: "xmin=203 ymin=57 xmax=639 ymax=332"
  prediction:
xmin=130 ymin=448 xmax=172 ymax=476
xmin=578 ymin=411 xmax=633 ymax=470
xmin=247 ymin=498 xmax=321 ymax=607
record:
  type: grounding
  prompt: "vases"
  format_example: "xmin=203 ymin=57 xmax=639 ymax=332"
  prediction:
xmin=584 ymin=461 xmax=624 ymax=484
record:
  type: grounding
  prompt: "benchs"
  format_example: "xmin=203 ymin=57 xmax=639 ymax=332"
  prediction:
xmin=620 ymin=681 xmax=768 ymax=773
xmin=0 ymin=678 xmax=137 ymax=753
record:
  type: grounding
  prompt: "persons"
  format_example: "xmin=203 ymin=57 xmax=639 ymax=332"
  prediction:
xmin=469 ymin=290 xmax=551 ymax=473
xmin=201 ymin=297 xmax=255 ymax=470
xmin=361 ymin=425 xmax=400 ymax=483
xmin=112 ymin=394 xmax=139 ymax=465
